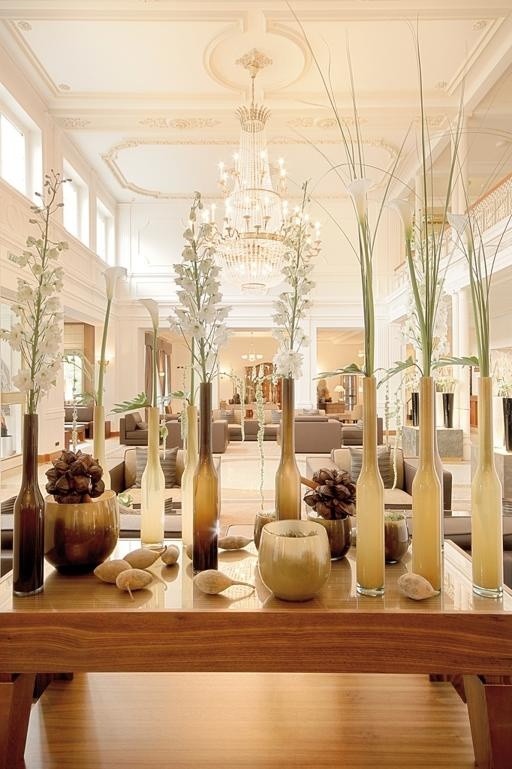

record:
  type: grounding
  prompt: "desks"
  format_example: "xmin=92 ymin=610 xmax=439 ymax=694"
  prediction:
xmin=0 ymin=540 xmax=512 ymax=767
